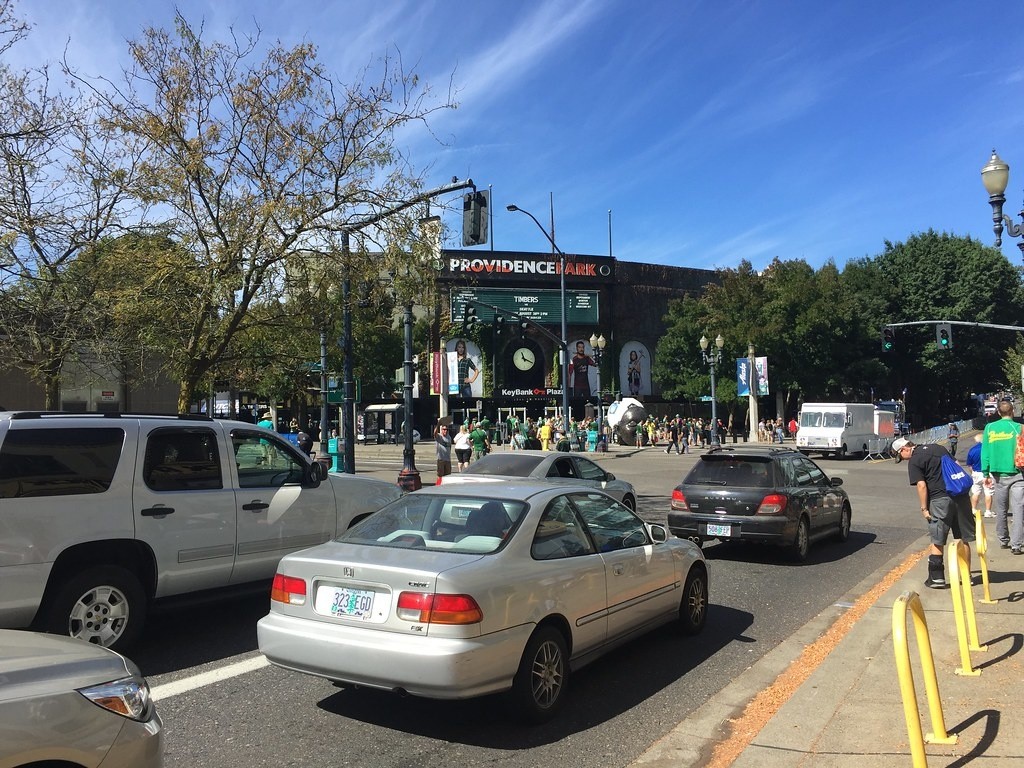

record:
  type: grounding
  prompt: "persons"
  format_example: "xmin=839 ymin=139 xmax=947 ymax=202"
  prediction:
xmin=505 ymin=414 xmax=609 ymax=452
xmin=891 ymin=438 xmax=977 ymax=589
xmin=453 ymin=425 xmax=474 ymax=472
xmin=463 ymin=416 xmax=490 ymax=441
xmin=434 ymin=421 xmax=451 ymax=477
xmin=297 ymin=428 xmax=310 ymax=457
xmin=466 ymin=422 xmax=490 ymax=462
xmin=948 ymin=422 xmax=960 ymax=458
xmin=981 ymin=401 xmax=1024 ymax=555
xmin=731 ymin=421 xmax=737 ymax=443
xmin=627 ymin=350 xmax=641 ymax=395
xmin=788 ymin=417 xmax=798 ymax=443
xmin=758 ymin=417 xmax=784 ymax=445
xmin=454 ymin=340 xmax=479 ymax=398
xmin=257 ymin=412 xmax=275 ymax=466
xmin=568 ymin=341 xmax=598 ymax=397
xmin=966 ymin=433 xmax=997 ymax=518
xmin=635 ymin=413 xmax=728 ymax=456
xmin=741 ymin=426 xmax=749 ymax=442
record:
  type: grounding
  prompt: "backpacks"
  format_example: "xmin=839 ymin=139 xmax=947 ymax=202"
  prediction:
xmin=913 ymin=443 xmax=973 ymax=498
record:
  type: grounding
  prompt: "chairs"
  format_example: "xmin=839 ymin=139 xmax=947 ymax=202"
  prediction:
xmin=376 ymin=530 xmax=502 ymax=553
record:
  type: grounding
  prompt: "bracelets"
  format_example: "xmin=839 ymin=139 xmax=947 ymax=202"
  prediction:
xmin=921 ymin=508 xmax=927 ymax=511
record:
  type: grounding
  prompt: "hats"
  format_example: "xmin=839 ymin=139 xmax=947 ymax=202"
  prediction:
xmin=890 ymin=437 xmax=910 ymax=464
xmin=474 ymin=422 xmax=482 ymax=428
xmin=262 ymin=412 xmax=272 ymax=419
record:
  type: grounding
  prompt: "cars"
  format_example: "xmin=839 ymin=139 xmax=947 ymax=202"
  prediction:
xmin=256 ymin=482 xmax=713 ymax=724
xmin=984 ymin=395 xmax=1013 ymax=417
xmin=433 ymin=449 xmax=638 ymax=542
xmin=0 ymin=626 xmax=167 ymax=768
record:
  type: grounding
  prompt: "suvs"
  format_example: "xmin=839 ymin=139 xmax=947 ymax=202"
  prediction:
xmin=667 ymin=443 xmax=852 ymax=562
xmin=0 ymin=408 xmax=410 ymax=664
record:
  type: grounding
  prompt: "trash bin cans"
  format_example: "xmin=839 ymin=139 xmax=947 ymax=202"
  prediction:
xmin=567 ymin=431 xmax=587 ymax=452
xmin=277 ymin=434 xmax=300 ymax=447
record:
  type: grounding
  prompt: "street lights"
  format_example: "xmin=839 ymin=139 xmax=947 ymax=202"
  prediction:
xmin=748 ymin=340 xmax=761 ymax=444
xmin=505 ymin=204 xmax=571 ymax=436
xmin=589 ymin=333 xmax=606 ymax=442
xmin=307 ymin=277 xmax=336 ymax=471
xmin=393 ymin=253 xmax=430 ymax=491
xmin=699 ymin=334 xmax=725 ymax=453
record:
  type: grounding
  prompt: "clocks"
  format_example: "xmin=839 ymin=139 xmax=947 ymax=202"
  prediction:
xmin=513 ymin=347 xmax=536 ymax=371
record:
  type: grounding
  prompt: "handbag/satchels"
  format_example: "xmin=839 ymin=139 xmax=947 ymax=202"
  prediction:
xmin=1015 ymin=434 xmax=1024 ymax=468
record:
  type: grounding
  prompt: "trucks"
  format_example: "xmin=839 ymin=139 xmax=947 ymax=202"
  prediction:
xmin=873 ymin=400 xmax=910 ymax=436
xmin=795 ymin=402 xmax=896 ymax=460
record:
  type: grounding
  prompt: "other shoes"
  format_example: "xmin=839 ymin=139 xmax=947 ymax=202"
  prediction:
xmin=984 ymin=512 xmax=996 ymax=518
xmin=1011 ymin=549 xmax=1021 ymax=554
xmin=969 ymin=575 xmax=975 ymax=585
xmin=925 ymin=578 xmax=945 ymax=588
xmin=1001 ymin=545 xmax=1007 ymax=549
xmin=664 ymin=450 xmax=668 ymax=454
xmin=973 ymin=510 xmax=976 ymax=515
xmin=676 ymin=453 xmax=679 ymax=455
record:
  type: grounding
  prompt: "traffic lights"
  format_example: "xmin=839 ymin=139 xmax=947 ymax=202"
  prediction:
xmin=882 ymin=326 xmax=895 ymax=353
xmin=517 ymin=319 xmax=530 ymax=344
xmin=492 ymin=314 xmax=505 ymax=338
xmin=463 ymin=304 xmax=476 ymax=337
xmin=936 ymin=324 xmax=952 ymax=351
